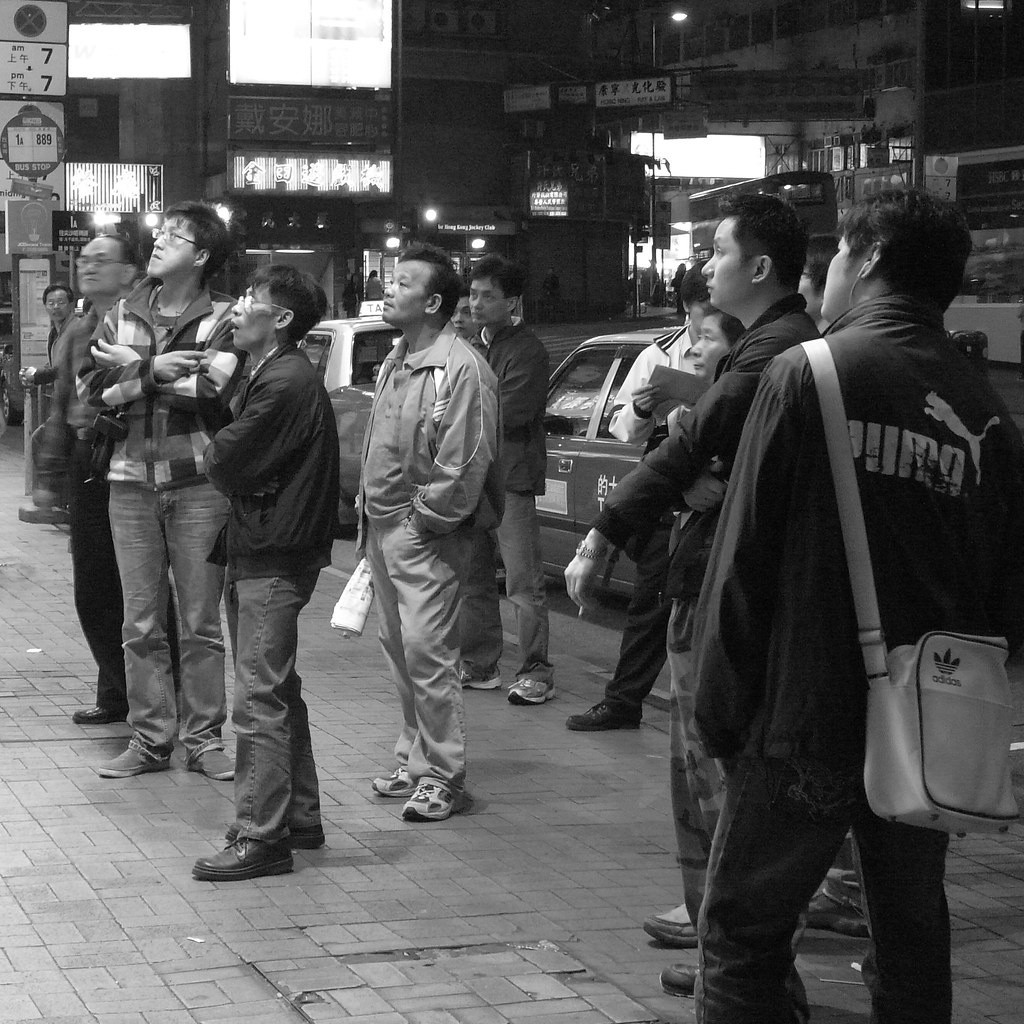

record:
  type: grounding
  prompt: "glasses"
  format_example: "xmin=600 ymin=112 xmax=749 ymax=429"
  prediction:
xmin=152 ymin=227 xmax=209 ymax=249
xmin=238 ymin=296 xmax=289 ymax=315
xmin=75 ymin=255 xmax=135 ymax=270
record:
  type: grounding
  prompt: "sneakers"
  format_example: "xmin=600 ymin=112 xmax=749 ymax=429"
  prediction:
xmin=644 ymin=902 xmax=699 ymax=950
xmin=659 ymin=964 xmax=704 ymax=999
xmin=401 ymin=784 xmax=454 ymax=821
xmin=566 ymin=699 xmax=640 ymax=731
xmin=449 ymin=657 xmax=502 ymax=690
xmin=373 ymin=768 xmax=422 ymax=797
xmin=188 ymin=747 xmax=235 ymax=779
xmin=507 ymin=678 xmax=555 ymax=705
xmin=795 ymin=886 xmax=870 ymax=939
xmin=98 ymin=746 xmax=171 ymax=778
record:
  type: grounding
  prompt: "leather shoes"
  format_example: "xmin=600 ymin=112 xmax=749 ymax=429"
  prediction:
xmin=224 ymin=821 xmax=326 ymax=849
xmin=191 ymin=835 xmax=294 ymax=881
xmin=72 ymin=704 xmax=129 ymax=725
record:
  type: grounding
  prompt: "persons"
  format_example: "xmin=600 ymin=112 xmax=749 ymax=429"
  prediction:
xmin=675 ymin=261 xmax=687 ymax=313
xmin=565 ymin=259 xmax=712 ymax=731
xmin=365 ymin=269 xmax=384 ymax=300
xmin=799 ymin=234 xmax=872 ymax=938
xmin=76 ymin=192 xmax=250 ymax=780
xmin=562 ymin=194 xmax=808 ymax=997
xmin=18 ymin=281 xmax=75 ymax=510
xmin=535 ymin=268 xmax=559 ymax=327
xmin=695 ymin=188 xmax=1023 ymax=1023
xmin=343 ymin=273 xmax=362 ymax=318
xmin=34 ymin=232 xmax=130 ymax=725
xmin=450 ymin=282 xmax=476 ymax=338
xmin=458 ymin=256 xmax=557 ymax=704
xmin=353 ymin=242 xmax=498 ymax=820
xmin=694 ymin=303 xmax=747 ymax=783
xmin=192 ymin=263 xmax=339 ymax=878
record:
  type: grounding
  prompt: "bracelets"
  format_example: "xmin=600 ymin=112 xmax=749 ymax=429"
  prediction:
xmin=576 ymin=539 xmax=606 ymax=559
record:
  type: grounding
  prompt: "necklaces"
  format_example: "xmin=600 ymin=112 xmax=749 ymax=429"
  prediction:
xmin=252 ymin=345 xmax=275 ymax=370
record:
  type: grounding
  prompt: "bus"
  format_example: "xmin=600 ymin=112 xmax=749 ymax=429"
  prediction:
xmin=833 ymin=146 xmax=1024 ymax=372
xmin=687 ymin=168 xmax=839 ymax=289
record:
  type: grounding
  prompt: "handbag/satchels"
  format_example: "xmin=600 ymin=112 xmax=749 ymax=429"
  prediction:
xmin=864 ymin=631 xmax=1019 ymax=838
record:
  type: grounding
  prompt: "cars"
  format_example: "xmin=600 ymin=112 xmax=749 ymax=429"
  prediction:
xmin=492 ymin=322 xmax=691 ymax=607
xmin=0 ymin=333 xmax=23 ymax=429
xmin=281 ymin=313 xmax=507 ymax=539
